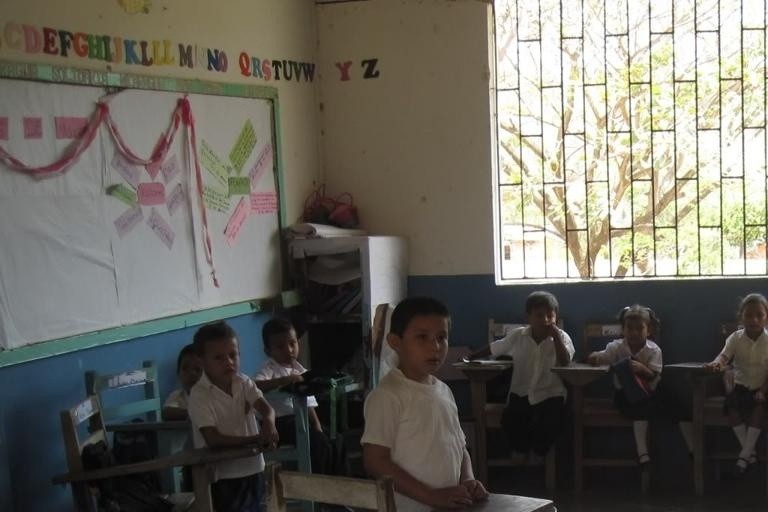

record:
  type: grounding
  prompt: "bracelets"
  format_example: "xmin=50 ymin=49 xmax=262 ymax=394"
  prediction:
xmin=554 ymin=329 xmax=562 ymax=343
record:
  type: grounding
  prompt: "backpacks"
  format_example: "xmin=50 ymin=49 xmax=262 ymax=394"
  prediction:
xmin=113 ymin=417 xmax=163 ymax=494
xmin=82 ymin=440 xmax=175 ymax=512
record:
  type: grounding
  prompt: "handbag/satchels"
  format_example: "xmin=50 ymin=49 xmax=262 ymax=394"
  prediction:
xmin=303 ymin=193 xmax=358 ymax=228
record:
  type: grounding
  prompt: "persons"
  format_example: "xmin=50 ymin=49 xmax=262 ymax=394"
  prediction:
xmin=700 ymin=292 xmax=767 ymax=479
xmin=186 ymin=320 xmax=280 ymax=510
xmin=466 ymin=289 xmax=577 ymax=466
xmin=160 ymin=346 xmax=206 ymax=420
xmin=358 ymin=294 xmax=490 ymax=512
xmin=250 ymin=308 xmax=335 ymax=475
xmin=588 ymin=299 xmax=697 ymax=474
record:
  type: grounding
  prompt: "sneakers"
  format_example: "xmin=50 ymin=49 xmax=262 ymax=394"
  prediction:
xmin=733 ymin=453 xmax=760 ymax=478
xmin=639 ymin=452 xmax=655 ymax=472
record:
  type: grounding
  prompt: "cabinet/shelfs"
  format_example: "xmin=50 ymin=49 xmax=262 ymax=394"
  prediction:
xmin=287 ymin=236 xmax=408 ymax=403
